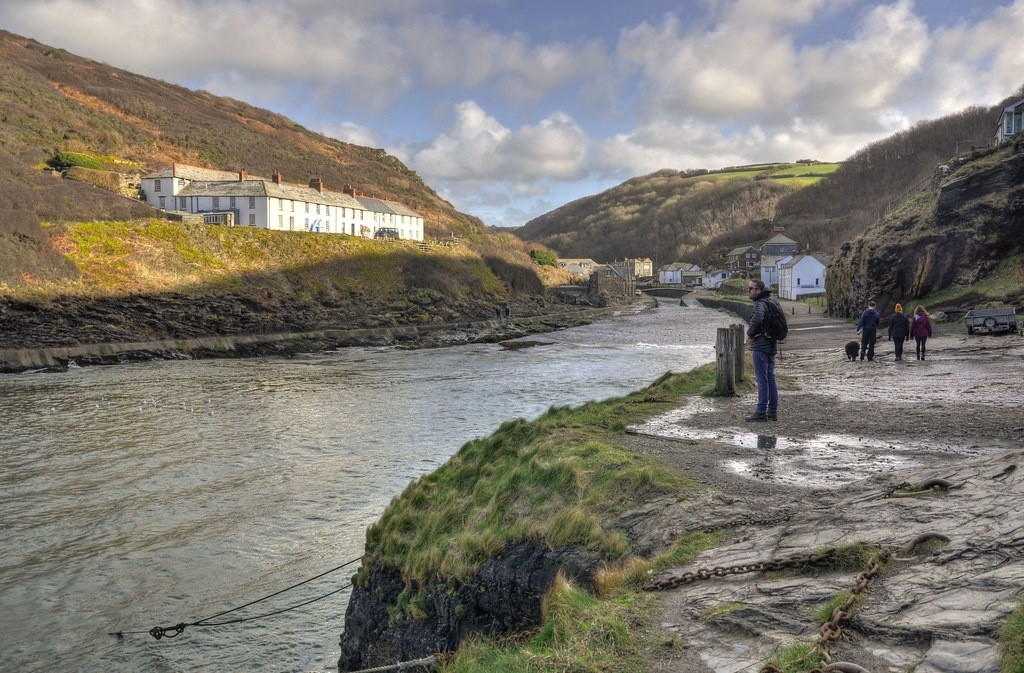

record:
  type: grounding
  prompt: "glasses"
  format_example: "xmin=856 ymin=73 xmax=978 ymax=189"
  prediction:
xmin=749 ymin=286 xmax=758 ymax=290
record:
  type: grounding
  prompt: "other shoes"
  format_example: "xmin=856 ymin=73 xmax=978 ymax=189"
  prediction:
xmin=917 ymin=357 xmax=920 ymax=360
xmin=868 ymin=358 xmax=874 ymax=361
xmin=895 ymin=355 xmax=902 ymax=361
xmin=860 ymin=354 xmax=864 ymax=359
xmin=922 ymin=358 xmax=925 ymax=360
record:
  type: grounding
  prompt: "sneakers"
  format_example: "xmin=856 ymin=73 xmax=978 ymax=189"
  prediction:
xmin=745 ymin=412 xmax=778 ymax=422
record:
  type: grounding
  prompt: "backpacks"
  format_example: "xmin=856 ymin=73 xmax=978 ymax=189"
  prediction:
xmin=759 ymin=300 xmax=788 ymax=341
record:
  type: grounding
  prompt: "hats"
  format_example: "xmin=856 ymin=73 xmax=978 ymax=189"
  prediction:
xmin=895 ymin=304 xmax=902 ymax=312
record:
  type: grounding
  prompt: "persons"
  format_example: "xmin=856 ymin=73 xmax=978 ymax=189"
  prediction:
xmin=910 ymin=305 xmax=932 ymax=360
xmin=746 ymin=279 xmax=779 ymax=420
xmin=888 ymin=303 xmax=910 ymax=361
xmin=857 ymin=301 xmax=880 ymax=362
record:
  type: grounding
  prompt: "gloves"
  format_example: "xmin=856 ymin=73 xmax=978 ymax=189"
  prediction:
xmin=889 ymin=336 xmax=890 ymax=341
xmin=906 ymin=337 xmax=909 ymax=341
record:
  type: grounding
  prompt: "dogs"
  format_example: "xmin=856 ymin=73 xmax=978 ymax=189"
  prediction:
xmin=845 ymin=341 xmax=860 ymax=361
